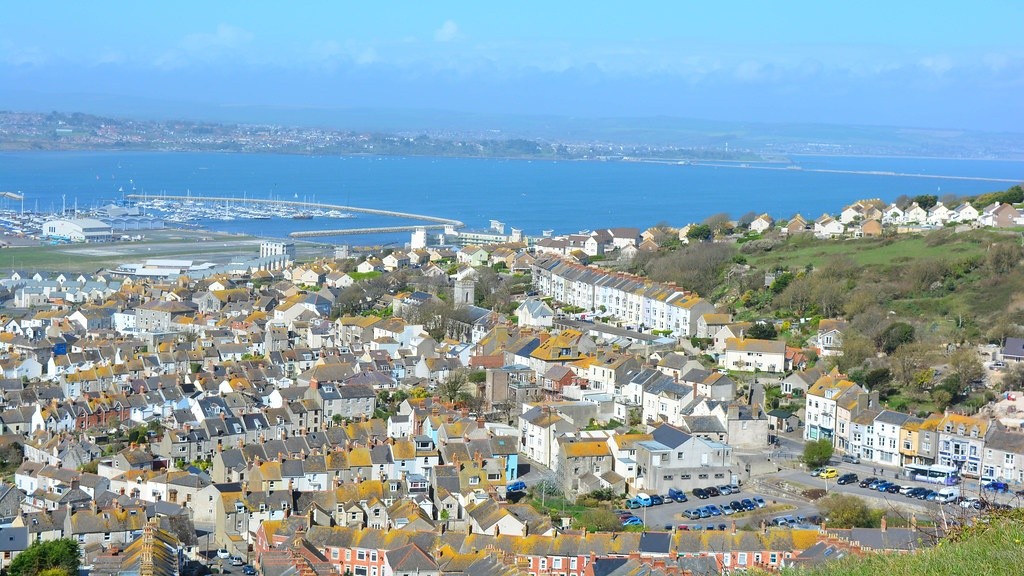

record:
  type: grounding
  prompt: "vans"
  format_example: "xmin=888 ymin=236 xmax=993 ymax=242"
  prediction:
xmin=682 ymin=509 xmax=700 ymax=520
xmin=669 ymin=488 xmax=687 ymax=502
xmin=637 ymin=493 xmax=652 ymax=506
xmin=934 ymin=486 xmax=961 ymax=505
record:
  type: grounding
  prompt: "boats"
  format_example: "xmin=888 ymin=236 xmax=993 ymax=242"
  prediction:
xmin=2 ymin=188 xmax=355 ymax=235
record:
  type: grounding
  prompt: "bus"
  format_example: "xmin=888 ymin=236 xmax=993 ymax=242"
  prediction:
xmin=903 ymin=464 xmax=960 ymax=486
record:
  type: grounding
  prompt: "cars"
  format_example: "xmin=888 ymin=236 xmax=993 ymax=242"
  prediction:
xmin=692 ymin=484 xmax=740 ymax=499
xmin=979 ymin=476 xmax=1010 ymax=492
xmin=650 ymin=494 xmax=672 ymax=505
xmin=626 ymin=499 xmax=640 ymax=509
xmin=706 ymin=505 xmax=721 ymax=516
xmin=742 ymin=499 xmax=754 ymax=511
xmin=753 ymin=496 xmax=766 ymax=508
xmin=859 ymin=477 xmax=938 ymax=501
xmin=1016 ymin=490 xmax=1024 ymax=499
xmin=811 ymin=468 xmax=838 ymax=479
xmin=719 ymin=503 xmax=735 ymax=515
xmin=613 ymin=509 xmax=643 ymax=527
xmin=665 ymin=523 xmax=727 ymax=532
xmin=507 ymin=481 xmax=527 ymax=492
xmin=730 ymin=501 xmax=746 ymax=511
xmin=147 ymin=430 xmax=158 ymax=438
xmin=837 ymin=473 xmax=858 ymax=485
xmin=697 ymin=507 xmax=709 ymax=518
xmin=842 ymin=455 xmax=860 ymax=464
xmin=217 ymin=548 xmax=255 ymax=576
xmin=959 ymin=497 xmax=1012 ymax=515
xmin=771 ymin=513 xmax=826 ymax=529
